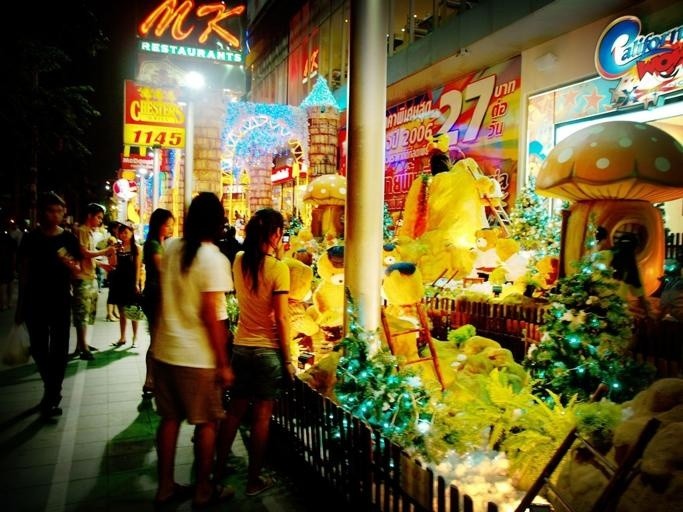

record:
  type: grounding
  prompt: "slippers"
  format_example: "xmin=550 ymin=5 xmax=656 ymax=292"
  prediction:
xmin=244 ymin=475 xmax=271 ymax=495
xmin=192 ymin=485 xmax=235 ymax=510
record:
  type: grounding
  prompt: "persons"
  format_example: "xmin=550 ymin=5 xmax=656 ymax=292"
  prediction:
xmin=140 ymin=207 xmax=183 ymax=399
xmin=0 ymin=196 xmax=148 ymax=362
xmin=11 ymin=189 xmax=84 ymax=418
xmin=211 ymin=206 xmax=298 ymax=495
xmin=143 ymin=192 xmax=240 ymax=509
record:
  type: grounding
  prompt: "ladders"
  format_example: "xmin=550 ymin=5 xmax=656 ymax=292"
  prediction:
xmin=382 ymin=299 xmax=449 ymax=393
xmin=516 ymin=382 xmax=662 ymax=512
xmin=419 ymin=268 xmax=459 ymax=307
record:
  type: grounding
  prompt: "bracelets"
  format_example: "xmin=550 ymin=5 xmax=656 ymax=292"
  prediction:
xmin=283 ymin=360 xmax=292 ymax=365
